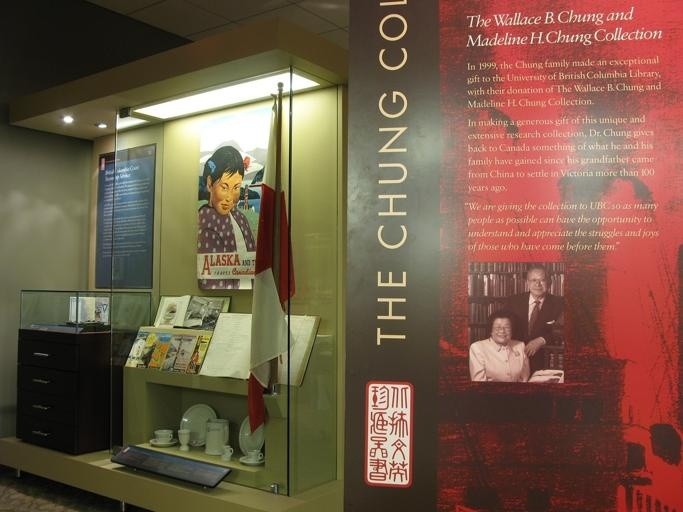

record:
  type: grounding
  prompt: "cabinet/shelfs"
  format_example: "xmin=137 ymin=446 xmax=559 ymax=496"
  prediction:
xmin=17 ymin=328 xmax=134 ymax=457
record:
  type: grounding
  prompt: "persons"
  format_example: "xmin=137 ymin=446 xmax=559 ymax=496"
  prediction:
xmin=469 ymin=309 xmax=530 ymax=382
xmin=505 ymin=266 xmax=560 ymax=372
xmin=198 ymin=146 xmax=256 ymax=289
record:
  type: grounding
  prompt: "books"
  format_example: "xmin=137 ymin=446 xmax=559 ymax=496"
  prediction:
xmin=125 ymin=294 xmax=223 ymax=374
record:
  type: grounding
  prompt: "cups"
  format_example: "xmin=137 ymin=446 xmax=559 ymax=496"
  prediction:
xmin=220 ymin=444 xmax=232 ymax=461
xmin=247 ymin=450 xmax=264 ymax=462
xmin=154 ymin=429 xmax=174 ymax=443
xmin=176 ymin=430 xmax=190 ymax=450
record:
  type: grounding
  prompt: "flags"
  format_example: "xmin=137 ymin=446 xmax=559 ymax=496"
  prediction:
xmin=248 ymin=94 xmax=295 ymax=432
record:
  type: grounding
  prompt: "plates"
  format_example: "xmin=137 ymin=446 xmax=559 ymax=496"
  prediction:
xmin=238 ymin=455 xmax=265 ymax=466
xmin=238 ymin=415 xmax=263 ymax=453
xmin=149 ymin=438 xmax=178 ymax=447
xmin=179 ymin=402 xmax=217 ymax=447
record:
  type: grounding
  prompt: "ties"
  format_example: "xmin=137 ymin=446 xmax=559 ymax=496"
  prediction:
xmin=529 ymin=300 xmax=541 ymax=334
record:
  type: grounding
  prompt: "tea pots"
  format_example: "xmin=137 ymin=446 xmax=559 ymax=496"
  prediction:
xmin=204 ymin=417 xmax=229 ymax=455
